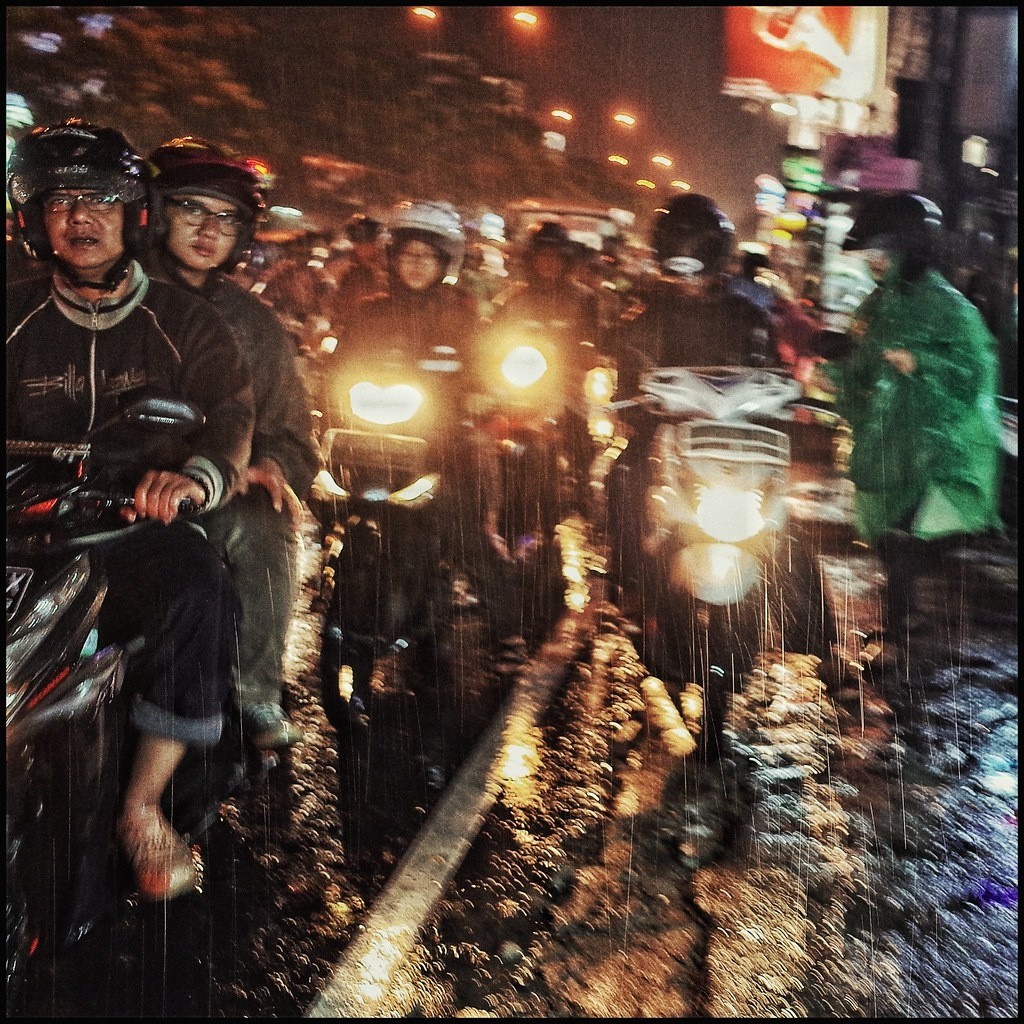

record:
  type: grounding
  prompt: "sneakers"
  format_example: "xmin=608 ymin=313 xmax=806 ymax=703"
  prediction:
xmin=234 ymin=693 xmax=299 ymax=750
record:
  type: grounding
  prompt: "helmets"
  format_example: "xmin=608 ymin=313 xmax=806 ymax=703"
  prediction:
xmin=6 ymin=116 xmax=152 ymax=261
xmin=376 ymin=199 xmax=465 ymax=279
xmin=521 ymin=220 xmax=575 ymax=294
xmin=652 ymin=195 xmax=734 ymax=277
xmin=841 ymin=193 xmax=949 ymax=292
xmin=144 ymin=133 xmax=268 ymax=274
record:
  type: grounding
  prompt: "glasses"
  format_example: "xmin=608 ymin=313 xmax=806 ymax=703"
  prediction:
xmin=42 ymin=193 xmax=117 ymax=213
xmin=400 ymin=250 xmax=443 ymax=265
xmin=165 ymin=196 xmax=249 ymax=236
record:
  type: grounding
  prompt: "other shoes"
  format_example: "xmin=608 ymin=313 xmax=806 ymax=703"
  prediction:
xmin=817 ymin=655 xmax=867 ymax=703
xmin=119 ymin=806 xmax=196 ymax=900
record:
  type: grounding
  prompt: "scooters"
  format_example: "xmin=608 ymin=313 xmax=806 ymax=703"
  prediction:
xmin=4 ymin=285 xmax=1021 ymax=1021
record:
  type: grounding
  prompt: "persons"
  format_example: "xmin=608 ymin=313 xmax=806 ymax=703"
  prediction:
xmin=4 ymin=121 xmax=1004 ymax=902
